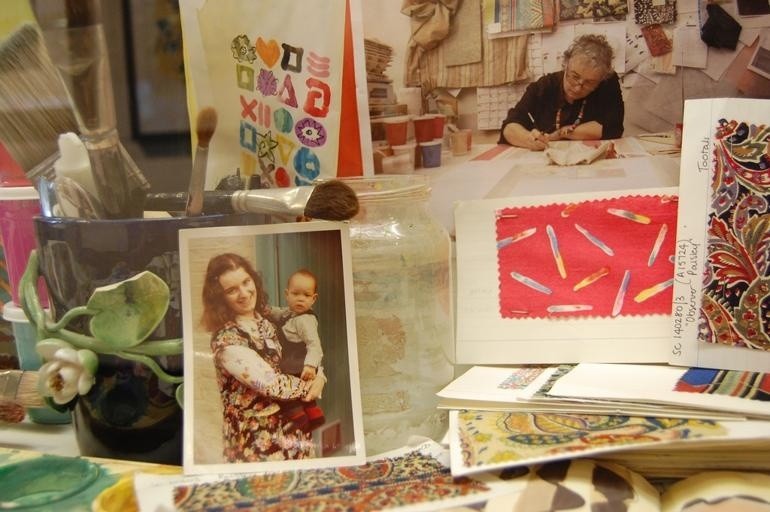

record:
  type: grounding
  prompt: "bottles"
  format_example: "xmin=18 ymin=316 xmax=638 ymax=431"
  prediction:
xmin=0 ymin=199 xmax=73 ymax=426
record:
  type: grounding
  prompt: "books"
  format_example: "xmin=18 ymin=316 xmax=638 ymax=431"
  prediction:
xmin=433 ymin=363 xmax=770 ymax=476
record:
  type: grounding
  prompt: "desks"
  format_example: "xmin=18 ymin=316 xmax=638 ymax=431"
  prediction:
xmin=361 ymin=129 xmax=684 ymax=246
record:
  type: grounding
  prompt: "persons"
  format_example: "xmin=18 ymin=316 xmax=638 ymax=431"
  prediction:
xmin=257 ymin=266 xmax=326 ymax=435
xmin=204 ymin=251 xmax=331 ymax=464
xmin=497 ymin=34 xmax=625 ymax=147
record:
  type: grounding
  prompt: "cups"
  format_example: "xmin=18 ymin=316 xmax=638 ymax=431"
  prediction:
xmin=18 ymin=211 xmax=272 ymax=466
xmin=370 ymin=113 xmax=472 ymax=175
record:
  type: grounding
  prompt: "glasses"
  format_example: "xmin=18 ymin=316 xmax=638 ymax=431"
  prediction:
xmin=566 ymin=60 xmax=599 ymax=92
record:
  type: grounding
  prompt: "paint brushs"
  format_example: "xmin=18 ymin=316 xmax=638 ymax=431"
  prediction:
xmin=38 ymin=0 xmax=359 ymax=220
xmin=0 ymin=371 xmax=45 ymax=409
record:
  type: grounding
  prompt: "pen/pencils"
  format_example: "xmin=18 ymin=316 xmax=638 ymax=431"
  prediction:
xmin=528 ymin=112 xmax=540 ymax=130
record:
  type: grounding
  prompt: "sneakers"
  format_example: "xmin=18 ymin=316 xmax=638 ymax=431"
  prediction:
xmin=300 ymin=415 xmax=325 ymax=434
xmin=282 ymin=413 xmax=307 ymax=435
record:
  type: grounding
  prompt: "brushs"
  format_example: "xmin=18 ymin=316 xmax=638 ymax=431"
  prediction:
xmin=0 ymin=24 xmax=82 ymax=218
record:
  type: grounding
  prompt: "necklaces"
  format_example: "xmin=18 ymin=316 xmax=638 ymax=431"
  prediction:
xmin=554 ymin=99 xmax=584 ymax=138
